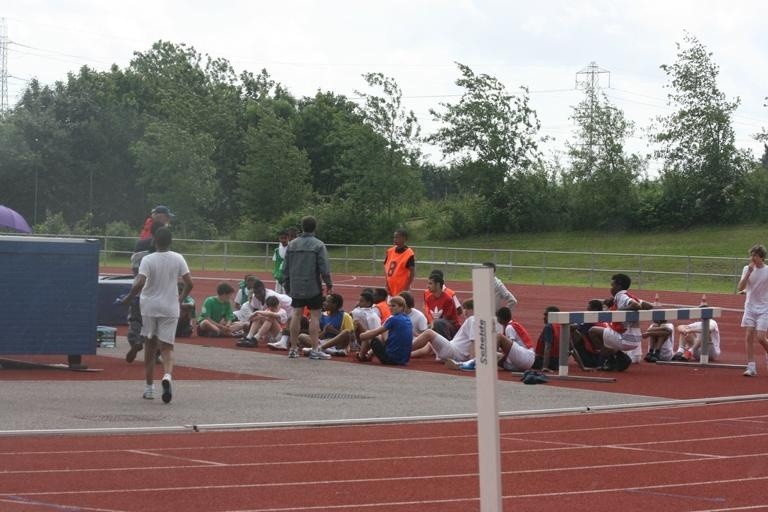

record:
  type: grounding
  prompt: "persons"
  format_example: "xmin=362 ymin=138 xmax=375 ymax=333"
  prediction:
xmin=252 ymin=281 xmax=294 ymax=318
xmin=151 ymin=206 xmax=176 ymax=222
xmin=383 ymin=230 xmax=416 ymax=304
xmin=482 ymin=263 xmax=518 ymax=315
xmin=126 ymin=240 xmax=161 ymax=363
xmin=353 ymin=288 xmax=373 ymax=309
xmin=233 ymin=300 xmax=255 ymax=321
xmin=390 ymin=291 xmax=427 ymax=341
xmin=738 ymin=244 xmax=768 ymax=376
xmin=140 ymin=209 xmax=156 ymax=239
xmin=356 ymin=296 xmax=413 ymax=365
xmin=135 ymin=221 xmax=165 ymax=252
xmin=282 ymin=216 xmax=332 ymax=360
xmin=531 ymin=306 xmax=561 ymax=374
xmin=424 ymin=270 xmax=462 ymax=318
xmin=233 ymin=276 xmax=257 ymax=310
xmin=410 ymin=299 xmax=475 ymax=363
xmin=589 ymin=274 xmax=653 ymax=366
xmin=236 ymin=296 xmax=288 ymax=347
xmin=267 ymin=313 xmax=309 ymax=350
xmin=445 ymin=307 xmax=535 ymax=372
xmin=675 ymin=304 xmax=720 ymax=361
xmin=569 ymin=300 xmax=613 ymax=366
xmin=271 ymin=231 xmax=290 ymax=294
xmin=195 ymin=283 xmax=236 ymax=337
xmin=642 ymin=321 xmax=675 ymax=362
xmin=349 ymin=293 xmax=381 ymax=350
xmin=298 ymin=294 xmax=354 ymax=357
xmin=425 ymin=274 xmax=457 ymax=324
xmin=177 ymin=282 xmax=195 ymax=337
xmin=601 ymin=298 xmax=624 ymax=333
xmin=121 ymin=228 xmax=193 ymax=404
xmin=372 ymin=288 xmax=391 ymax=326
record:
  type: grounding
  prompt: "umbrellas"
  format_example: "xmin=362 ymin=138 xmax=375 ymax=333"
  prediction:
xmin=0 ymin=205 xmax=32 ymax=233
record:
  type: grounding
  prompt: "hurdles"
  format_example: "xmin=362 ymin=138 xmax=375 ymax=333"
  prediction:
xmin=511 ymin=307 xmax=748 ymax=382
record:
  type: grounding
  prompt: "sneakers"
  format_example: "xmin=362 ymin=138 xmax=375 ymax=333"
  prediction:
xmin=143 ymin=390 xmax=154 ymax=399
xmin=126 ymin=342 xmax=143 ymax=362
xmin=596 ymin=356 xmax=610 ymax=369
xmin=239 ymin=336 xmax=374 ymax=363
xmin=645 ymin=350 xmax=696 ymax=359
xmin=743 ymin=367 xmax=757 ymax=376
xmin=162 ymin=377 xmax=172 ymax=402
xmin=615 ymin=356 xmax=631 ymax=371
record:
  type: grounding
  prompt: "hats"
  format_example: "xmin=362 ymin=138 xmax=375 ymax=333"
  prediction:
xmin=151 ymin=205 xmax=175 ymax=217
